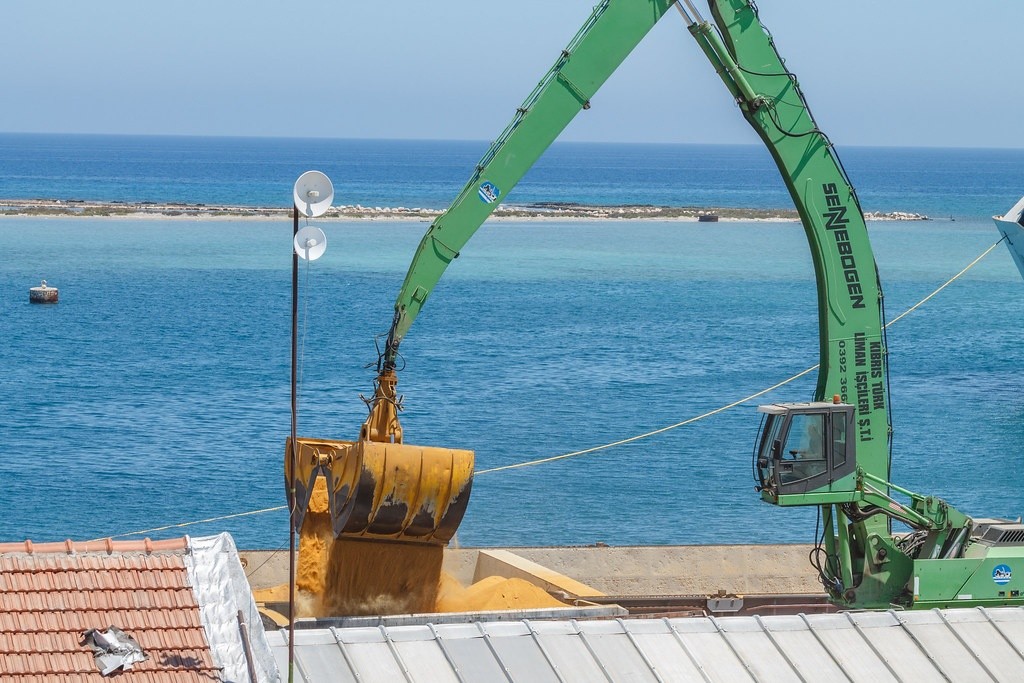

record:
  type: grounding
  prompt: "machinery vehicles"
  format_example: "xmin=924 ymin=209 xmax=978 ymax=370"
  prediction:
xmin=281 ymin=0 xmax=1024 ymax=609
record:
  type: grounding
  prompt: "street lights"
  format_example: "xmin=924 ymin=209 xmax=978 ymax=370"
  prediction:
xmin=288 ymin=169 xmax=335 ymax=683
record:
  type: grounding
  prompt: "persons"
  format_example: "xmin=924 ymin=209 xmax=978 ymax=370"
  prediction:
xmin=797 ymin=424 xmax=822 ymax=458
